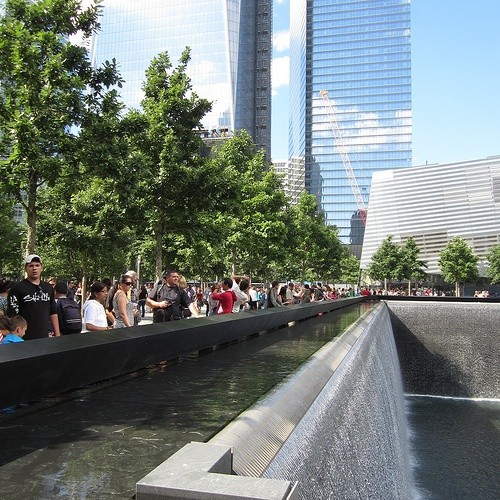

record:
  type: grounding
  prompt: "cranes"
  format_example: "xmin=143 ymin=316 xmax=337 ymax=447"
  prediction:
xmin=318 ymin=88 xmax=367 ymax=226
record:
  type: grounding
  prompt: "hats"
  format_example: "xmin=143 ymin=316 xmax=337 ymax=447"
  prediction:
xmin=25 ymin=254 xmax=42 ymax=263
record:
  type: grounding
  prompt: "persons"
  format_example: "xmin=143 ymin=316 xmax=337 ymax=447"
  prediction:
xmin=83 ymin=280 xmax=114 ymax=333
xmin=66 ymin=272 xmax=490 ymax=317
xmin=48 ymin=323 xmax=55 ymax=338
xmin=0 ymin=315 xmax=28 ymax=345
xmin=0 ymin=280 xmax=14 ymax=313
xmin=7 ymin=254 xmax=61 ymax=341
xmin=0 ymin=307 xmax=5 ymax=316
xmin=112 ymin=274 xmax=134 ymax=328
xmin=53 ymin=282 xmax=74 ymax=336
xmin=106 ymin=270 xmax=138 ymax=327
xmin=0 ymin=315 xmax=15 ymax=342
xmin=47 ymin=277 xmax=59 ymax=289
xmin=144 ymin=270 xmax=201 ymax=323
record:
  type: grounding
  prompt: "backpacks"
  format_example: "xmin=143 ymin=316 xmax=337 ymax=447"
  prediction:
xmin=56 ymin=298 xmax=82 ymax=334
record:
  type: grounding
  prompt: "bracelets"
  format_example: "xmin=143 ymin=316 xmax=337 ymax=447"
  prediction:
xmin=107 ymin=327 xmax=109 ymax=330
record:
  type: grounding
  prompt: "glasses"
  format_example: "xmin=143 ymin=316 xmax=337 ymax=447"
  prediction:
xmin=123 ymin=282 xmax=132 ymax=285
xmin=100 ymin=290 xmax=107 ymax=293
